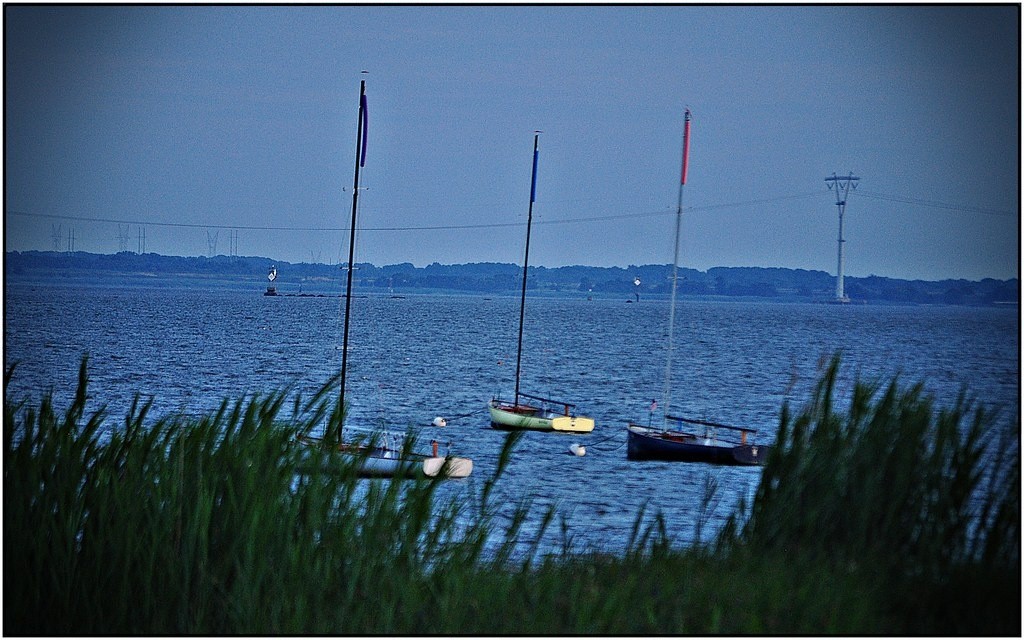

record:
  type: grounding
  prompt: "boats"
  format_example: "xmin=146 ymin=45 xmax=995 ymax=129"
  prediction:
xmin=486 ymin=130 xmax=596 ymax=432
xmin=291 ymin=80 xmax=475 ymax=477
xmin=626 ymin=106 xmax=774 ymax=466
